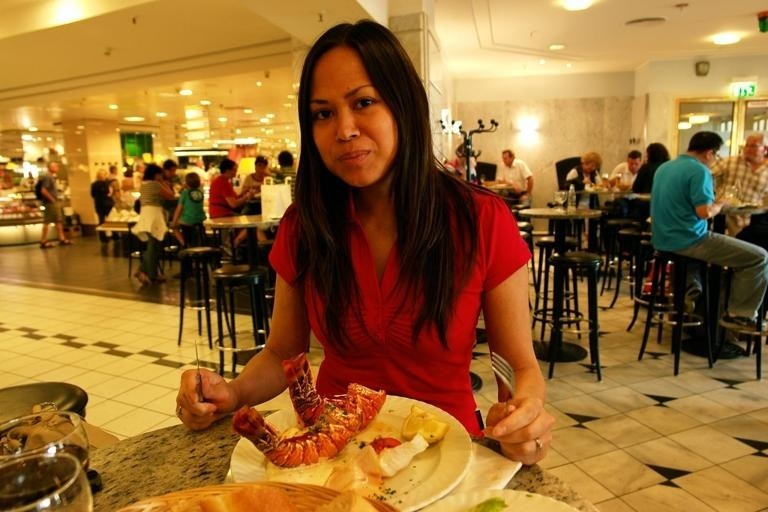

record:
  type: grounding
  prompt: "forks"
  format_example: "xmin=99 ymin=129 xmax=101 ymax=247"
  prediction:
xmin=491 ymin=347 xmax=517 ymax=404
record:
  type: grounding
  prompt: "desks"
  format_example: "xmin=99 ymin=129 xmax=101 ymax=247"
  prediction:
xmin=203 ymin=214 xmax=283 ymax=366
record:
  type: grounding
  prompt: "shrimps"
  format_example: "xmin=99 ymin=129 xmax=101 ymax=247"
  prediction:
xmin=281 ymin=352 xmax=387 ymax=438
xmin=232 ymin=394 xmax=363 ymax=468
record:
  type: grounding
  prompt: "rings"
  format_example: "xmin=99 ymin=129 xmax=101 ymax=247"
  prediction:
xmin=178 ymin=403 xmax=182 ymax=418
xmin=535 ymin=438 xmax=544 ymax=452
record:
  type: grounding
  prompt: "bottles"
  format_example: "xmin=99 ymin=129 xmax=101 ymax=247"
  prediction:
xmin=567 ymin=183 xmax=578 ymax=210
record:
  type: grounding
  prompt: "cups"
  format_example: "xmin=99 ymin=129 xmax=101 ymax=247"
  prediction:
xmin=1 ymin=412 xmax=91 ymax=471
xmin=0 ymin=454 xmax=94 ymax=512
xmin=724 ymin=185 xmax=737 ymax=200
xmin=600 ymin=173 xmax=609 ymax=189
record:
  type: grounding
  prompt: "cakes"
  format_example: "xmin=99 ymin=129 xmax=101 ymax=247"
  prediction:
xmin=118 ymin=480 xmax=381 ymax=512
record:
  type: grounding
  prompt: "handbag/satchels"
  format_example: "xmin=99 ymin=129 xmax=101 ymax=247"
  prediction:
xmin=261 ymin=175 xmax=292 ymax=219
xmin=35 ymin=180 xmax=45 ymax=202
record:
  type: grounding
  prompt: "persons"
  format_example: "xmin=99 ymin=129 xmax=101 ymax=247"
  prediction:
xmin=37 ymin=161 xmax=76 ymax=249
xmin=442 ymin=142 xmax=478 ymax=181
xmin=176 ymin=19 xmax=556 ymax=468
xmin=649 ymin=131 xmax=768 ymax=332
xmin=90 ymin=151 xmax=297 ymax=292
xmin=563 ymin=141 xmax=671 ymax=261
xmin=709 ymin=131 xmax=768 ymax=241
xmin=496 ymin=149 xmax=533 ymax=208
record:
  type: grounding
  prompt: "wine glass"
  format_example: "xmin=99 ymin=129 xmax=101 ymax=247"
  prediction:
xmin=554 ymin=190 xmax=568 ymax=213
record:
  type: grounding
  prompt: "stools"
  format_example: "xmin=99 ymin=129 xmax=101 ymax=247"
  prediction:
xmin=1 ymin=382 xmax=88 ymax=429
xmin=178 ymin=245 xmax=234 ymax=351
xmin=80 ymin=409 xmax=600 ymax=512
xmin=211 ymin=264 xmax=271 ymax=378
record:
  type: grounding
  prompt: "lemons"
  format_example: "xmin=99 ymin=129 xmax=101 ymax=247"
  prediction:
xmin=402 ymin=404 xmax=450 ymax=445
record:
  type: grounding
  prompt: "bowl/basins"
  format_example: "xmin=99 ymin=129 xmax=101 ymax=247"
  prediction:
xmin=617 ymin=184 xmax=630 ymax=193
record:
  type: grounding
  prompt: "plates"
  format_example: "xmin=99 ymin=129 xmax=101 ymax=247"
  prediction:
xmin=421 ymin=490 xmax=580 ymax=512
xmin=230 ymin=396 xmax=474 ymax=512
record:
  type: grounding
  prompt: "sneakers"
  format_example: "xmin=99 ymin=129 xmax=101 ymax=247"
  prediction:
xmin=136 ymin=272 xmax=153 ymax=286
xmin=720 ymin=309 xmax=765 ymax=331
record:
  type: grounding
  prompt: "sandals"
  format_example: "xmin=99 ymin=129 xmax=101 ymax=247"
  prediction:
xmin=60 ymin=239 xmax=72 ymax=244
xmin=40 ymin=240 xmax=55 ymax=248
